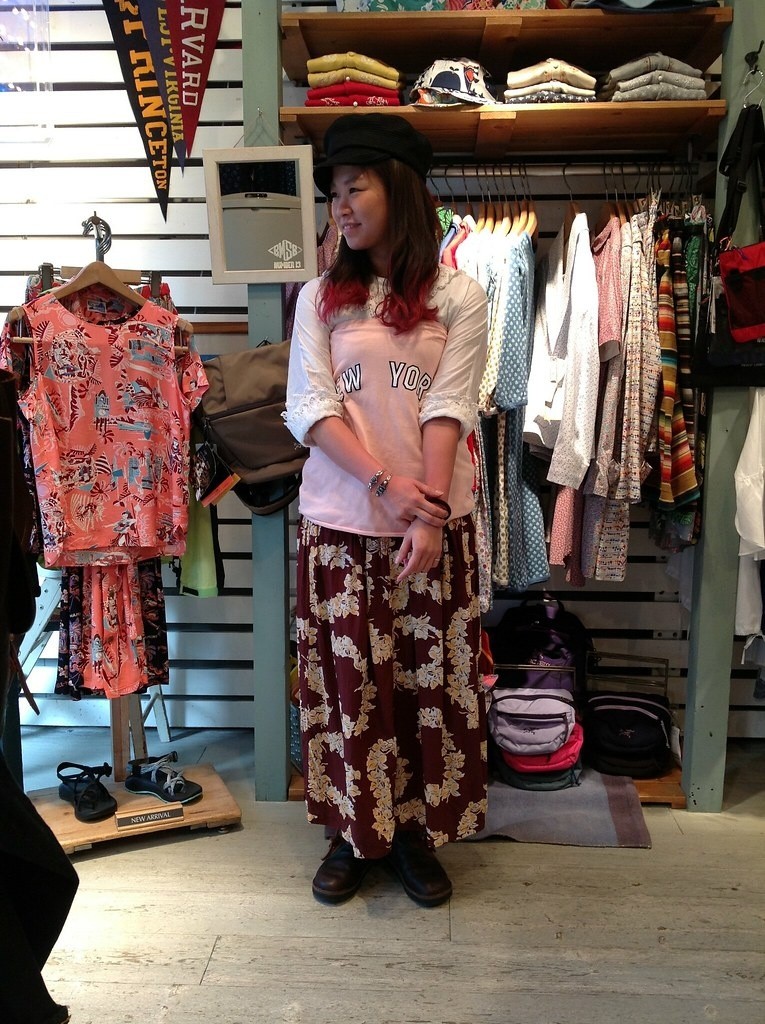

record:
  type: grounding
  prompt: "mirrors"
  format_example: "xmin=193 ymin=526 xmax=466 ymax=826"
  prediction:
xmin=202 ymin=145 xmax=318 ymax=285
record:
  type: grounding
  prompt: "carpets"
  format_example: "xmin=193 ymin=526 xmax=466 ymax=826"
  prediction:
xmin=325 ymin=764 xmax=652 ymax=847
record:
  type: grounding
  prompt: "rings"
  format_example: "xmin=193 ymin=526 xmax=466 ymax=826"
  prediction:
xmin=435 ymin=557 xmax=441 ymax=562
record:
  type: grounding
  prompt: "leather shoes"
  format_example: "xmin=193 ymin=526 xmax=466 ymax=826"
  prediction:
xmin=393 ymin=848 xmax=451 ymax=900
xmin=311 ymin=844 xmax=372 ymax=895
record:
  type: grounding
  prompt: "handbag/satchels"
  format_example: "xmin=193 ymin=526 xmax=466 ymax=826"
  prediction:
xmin=487 ymin=687 xmax=673 ymax=791
xmin=690 ymin=104 xmax=764 ymax=390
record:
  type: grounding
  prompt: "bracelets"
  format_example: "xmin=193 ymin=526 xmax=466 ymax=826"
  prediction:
xmin=367 ymin=468 xmax=393 ymax=497
xmin=424 ymin=494 xmax=451 ymax=521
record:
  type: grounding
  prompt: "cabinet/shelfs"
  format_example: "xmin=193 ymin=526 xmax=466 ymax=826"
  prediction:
xmin=240 ymin=0 xmax=765 ymax=814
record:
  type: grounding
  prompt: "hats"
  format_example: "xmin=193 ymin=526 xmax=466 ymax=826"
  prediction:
xmin=312 ymin=113 xmax=432 ymax=197
xmin=408 ymin=59 xmax=500 ymax=105
xmin=410 ymin=89 xmax=465 ymax=108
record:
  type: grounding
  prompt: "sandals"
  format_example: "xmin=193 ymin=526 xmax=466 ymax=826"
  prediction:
xmin=56 ymin=762 xmax=116 ymax=821
xmin=123 ymin=751 xmax=202 ymax=804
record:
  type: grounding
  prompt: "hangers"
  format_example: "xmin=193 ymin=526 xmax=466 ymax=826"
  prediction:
xmin=563 ymin=162 xmax=580 ymax=225
xmin=326 ymin=195 xmax=335 ymax=226
xmin=596 ymin=159 xmax=703 ymax=236
xmin=6 ymin=217 xmax=194 ymax=355
xmin=429 ymin=166 xmax=538 ymax=253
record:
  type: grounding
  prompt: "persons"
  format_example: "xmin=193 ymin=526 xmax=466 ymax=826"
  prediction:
xmin=278 ymin=112 xmax=491 ymax=909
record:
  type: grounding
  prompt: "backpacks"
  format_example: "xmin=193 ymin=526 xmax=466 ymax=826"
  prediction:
xmin=193 ymin=339 xmax=307 ymax=516
